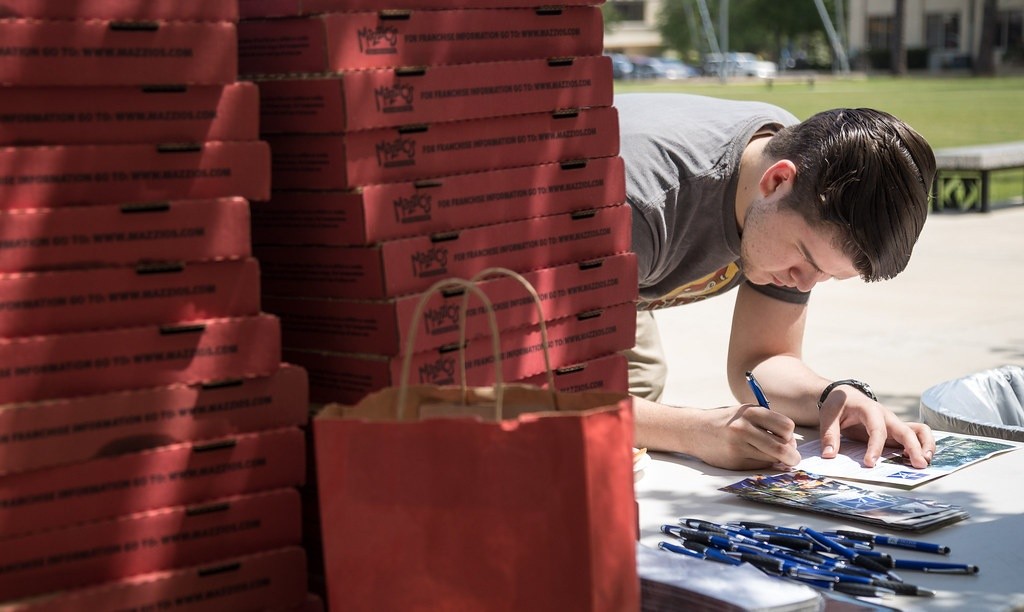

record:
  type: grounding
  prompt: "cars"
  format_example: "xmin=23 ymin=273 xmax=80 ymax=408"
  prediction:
xmin=706 ymin=52 xmax=778 ymax=79
xmin=614 ymin=57 xmax=697 ymax=80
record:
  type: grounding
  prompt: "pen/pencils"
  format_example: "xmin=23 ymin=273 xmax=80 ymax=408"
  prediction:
xmin=744 ymin=370 xmax=771 ymax=410
xmin=657 ymin=516 xmax=979 ymax=598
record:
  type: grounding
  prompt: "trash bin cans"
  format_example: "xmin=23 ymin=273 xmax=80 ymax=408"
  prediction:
xmin=921 ymin=363 xmax=1024 ymax=442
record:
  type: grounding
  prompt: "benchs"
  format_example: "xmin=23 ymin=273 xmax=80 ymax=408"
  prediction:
xmin=932 ymin=140 xmax=1024 ymax=214
xmin=765 ymin=75 xmax=819 ymax=90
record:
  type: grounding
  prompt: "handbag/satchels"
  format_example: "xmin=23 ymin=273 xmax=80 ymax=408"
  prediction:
xmin=313 ymin=266 xmax=643 ymax=612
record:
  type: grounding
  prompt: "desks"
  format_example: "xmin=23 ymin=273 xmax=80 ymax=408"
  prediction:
xmin=633 ymin=430 xmax=1024 ymax=612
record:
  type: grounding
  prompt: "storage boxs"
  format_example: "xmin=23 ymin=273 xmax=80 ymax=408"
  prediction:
xmin=0 ymin=0 xmax=317 ymax=612
xmin=514 ymin=352 xmax=629 ymax=395
xmin=283 ymin=301 xmax=638 ymax=404
xmin=254 ymin=54 xmax=614 ymax=132
xmin=265 ymin=106 xmax=622 ymax=193
xmin=252 ymin=156 xmax=627 ymax=241
xmin=279 ymin=252 xmax=637 ymax=361
xmin=240 ymin=6 xmax=607 ymax=73
xmin=257 ymin=203 xmax=634 ymax=299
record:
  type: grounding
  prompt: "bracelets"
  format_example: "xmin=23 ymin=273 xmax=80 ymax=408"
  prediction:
xmin=817 ymin=379 xmax=878 ymax=410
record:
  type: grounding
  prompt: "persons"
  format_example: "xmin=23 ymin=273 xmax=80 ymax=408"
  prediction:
xmin=613 ymin=92 xmax=937 ymax=470
